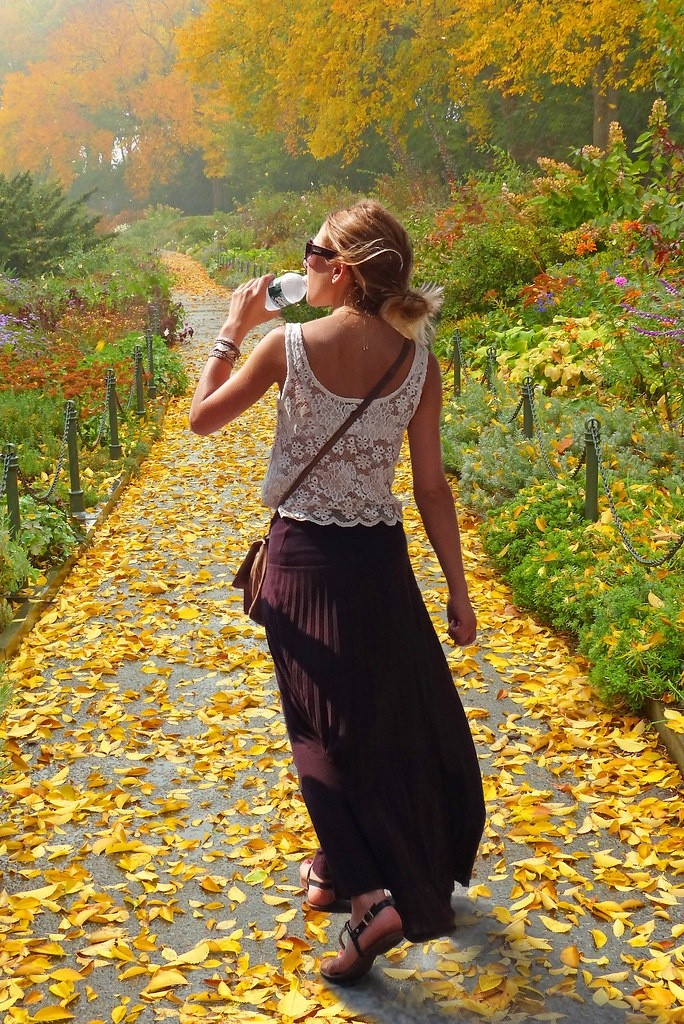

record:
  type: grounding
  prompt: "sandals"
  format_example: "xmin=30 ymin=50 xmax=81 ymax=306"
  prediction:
xmin=320 ymin=899 xmax=404 ymax=987
xmin=300 ymin=859 xmax=336 ymax=911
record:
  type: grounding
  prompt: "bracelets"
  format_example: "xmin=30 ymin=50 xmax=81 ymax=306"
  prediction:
xmin=207 ymin=337 xmax=242 ymax=368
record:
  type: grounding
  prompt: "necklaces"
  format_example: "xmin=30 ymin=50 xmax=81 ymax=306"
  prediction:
xmin=333 ymin=309 xmax=375 ymax=351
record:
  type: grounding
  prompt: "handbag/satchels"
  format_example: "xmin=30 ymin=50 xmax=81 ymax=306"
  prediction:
xmin=232 ymin=533 xmax=270 ymax=627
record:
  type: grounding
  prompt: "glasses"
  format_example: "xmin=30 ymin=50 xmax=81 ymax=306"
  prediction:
xmin=304 ymin=239 xmax=340 ymax=259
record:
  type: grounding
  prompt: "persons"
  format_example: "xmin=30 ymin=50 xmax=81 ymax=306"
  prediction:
xmin=186 ymin=200 xmax=487 ymax=983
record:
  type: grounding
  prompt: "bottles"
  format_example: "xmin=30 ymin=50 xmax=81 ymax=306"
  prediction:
xmin=265 ymin=273 xmax=308 ymax=311
